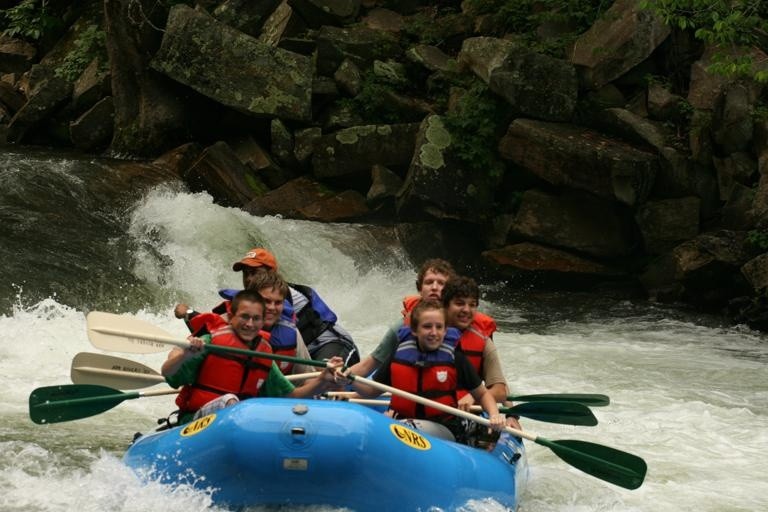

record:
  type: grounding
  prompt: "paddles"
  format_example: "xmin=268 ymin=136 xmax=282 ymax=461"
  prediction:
xmin=322 ymin=393 xmax=611 ymax=406
xmin=70 ymin=352 xmax=168 ymax=390
xmin=333 ymin=395 xmax=597 ymax=426
xmin=30 ymin=372 xmax=331 ymax=424
xmin=341 ymin=366 xmax=647 ymax=490
xmin=86 ymin=310 xmax=341 ymax=374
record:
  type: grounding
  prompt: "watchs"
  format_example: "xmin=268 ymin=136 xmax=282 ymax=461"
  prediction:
xmin=186 ymin=308 xmax=194 ymax=320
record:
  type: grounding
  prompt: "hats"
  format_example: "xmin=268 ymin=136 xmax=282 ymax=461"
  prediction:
xmin=233 ymin=249 xmax=276 ymax=271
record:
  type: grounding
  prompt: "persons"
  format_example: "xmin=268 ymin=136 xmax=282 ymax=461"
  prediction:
xmin=399 ymin=276 xmax=506 ymax=412
xmin=174 ymin=249 xmax=360 ymax=368
xmin=221 ymin=272 xmax=343 ymax=388
xmin=161 ymin=289 xmax=343 ymax=425
xmin=343 ymin=258 xmax=523 ymax=430
xmin=335 ymin=299 xmax=506 ymax=433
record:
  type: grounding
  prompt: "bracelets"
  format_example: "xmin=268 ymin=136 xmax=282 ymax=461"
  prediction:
xmin=506 ymin=413 xmax=520 ymax=420
xmin=348 ymin=380 xmax=354 ymax=386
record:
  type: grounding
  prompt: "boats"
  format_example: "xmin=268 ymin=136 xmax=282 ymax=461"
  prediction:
xmin=118 ymin=367 xmax=532 ymax=512
